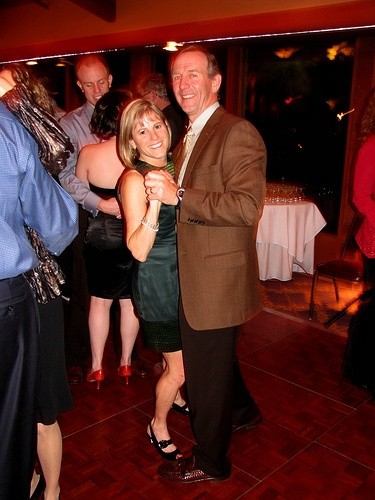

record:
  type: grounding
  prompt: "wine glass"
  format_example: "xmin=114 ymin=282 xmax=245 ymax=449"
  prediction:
xmin=264 ymin=183 xmax=304 ymax=205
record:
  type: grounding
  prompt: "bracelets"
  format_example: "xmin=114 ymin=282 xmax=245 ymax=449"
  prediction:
xmin=142 ymin=218 xmax=159 ymax=232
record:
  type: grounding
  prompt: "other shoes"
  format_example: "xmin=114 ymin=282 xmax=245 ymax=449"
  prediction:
xmin=69 ymin=367 xmax=82 ymax=384
xmin=133 ymin=361 xmax=148 ymax=378
xmin=355 ymin=380 xmax=375 ymax=391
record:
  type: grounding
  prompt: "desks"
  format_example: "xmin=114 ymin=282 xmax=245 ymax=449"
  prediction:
xmin=256 ymin=202 xmax=327 ymax=281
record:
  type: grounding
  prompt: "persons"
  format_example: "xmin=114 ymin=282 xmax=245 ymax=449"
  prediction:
xmin=0 ymin=61 xmax=75 ymax=500
xmin=57 ymin=54 xmax=186 ymax=390
xmin=144 ymin=41 xmax=267 ymax=483
xmin=342 ymin=86 xmax=375 ymax=398
xmin=0 ymin=100 xmax=81 ymax=500
xmin=117 ymin=99 xmax=190 ymax=460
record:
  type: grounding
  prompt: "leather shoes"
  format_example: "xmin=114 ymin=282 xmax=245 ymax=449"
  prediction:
xmin=231 ymin=413 xmax=263 ymax=433
xmin=157 ymin=454 xmax=232 ymax=484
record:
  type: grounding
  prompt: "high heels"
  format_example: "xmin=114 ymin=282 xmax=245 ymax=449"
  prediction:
xmin=115 ymin=365 xmax=132 ymax=385
xmin=30 ymin=473 xmax=45 ymax=500
xmin=146 ymin=422 xmax=184 ymax=462
xmin=86 ymin=369 xmax=105 ymax=390
xmin=172 ymin=400 xmax=190 ymax=417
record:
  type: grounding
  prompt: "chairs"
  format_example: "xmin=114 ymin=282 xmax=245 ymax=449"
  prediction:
xmin=308 ymin=213 xmax=365 ymax=322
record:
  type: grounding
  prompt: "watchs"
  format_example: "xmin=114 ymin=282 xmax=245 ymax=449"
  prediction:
xmin=175 ymin=187 xmax=185 ymax=209
